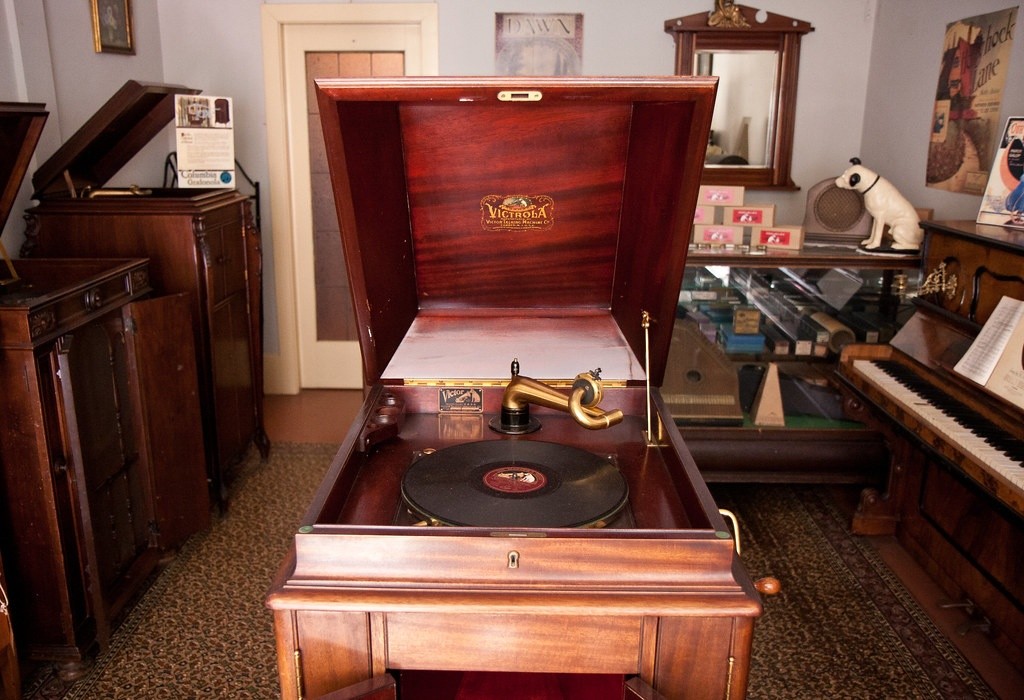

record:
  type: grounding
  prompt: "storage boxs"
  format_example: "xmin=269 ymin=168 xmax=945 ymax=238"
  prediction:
xmin=722 ymin=203 xmax=776 ymax=228
xmin=750 ymin=224 xmax=805 ymax=251
xmin=697 ymin=184 xmax=745 ymax=207
xmin=656 ymin=243 xmax=921 ymax=488
xmin=692 ymin=206 xmax=716 ymax=225
xmin=693 ymin=224 xmax=744 ymax=248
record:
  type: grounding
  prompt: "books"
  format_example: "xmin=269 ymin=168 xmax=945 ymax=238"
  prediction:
xmin=953 ymin=291 xmax=1024 ymax=408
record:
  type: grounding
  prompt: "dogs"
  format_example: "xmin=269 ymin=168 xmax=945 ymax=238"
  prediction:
xmin=834 ymin=157 xmax=925 ymax=250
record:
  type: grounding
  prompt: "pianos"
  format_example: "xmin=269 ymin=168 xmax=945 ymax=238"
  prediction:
xmin=831 ymin=296 xmax=1024 ymax=699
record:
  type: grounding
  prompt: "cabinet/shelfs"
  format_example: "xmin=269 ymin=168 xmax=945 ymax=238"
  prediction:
xmin=264 ymin=71 xmax=760 ymax=700
xmin=0 ymin=99 xmax=211 ymax=681
xmin=22 ymin=79 xmax=272 ymax=518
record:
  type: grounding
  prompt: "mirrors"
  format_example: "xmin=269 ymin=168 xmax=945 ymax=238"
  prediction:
xmin=663 ymin=0 xmax=817 ymax=190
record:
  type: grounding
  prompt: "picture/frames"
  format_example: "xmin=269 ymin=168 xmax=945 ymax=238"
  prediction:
xmin=492 ymin=11 xmax=584 ymax=77
xmin=974 ymin=116 xmax=1024 ymax=231
xmin=90 ymin=1 xmax=138 ymax=57
